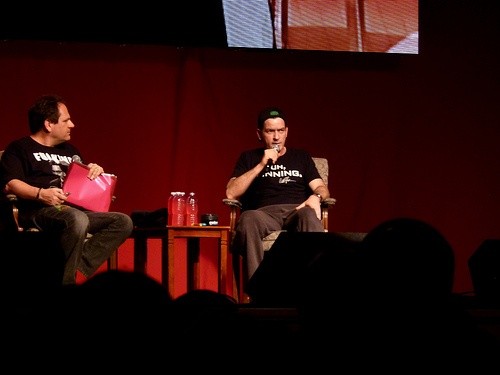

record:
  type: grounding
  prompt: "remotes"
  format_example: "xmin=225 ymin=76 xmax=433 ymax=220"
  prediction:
xmin=64 ymin=192 xmax=72 ymax=197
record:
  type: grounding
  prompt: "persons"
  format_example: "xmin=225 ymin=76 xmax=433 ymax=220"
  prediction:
xmin=225 ymin=107 xmax=336 ymax=281
xmin=0 ymin=94 xmax=133 ymax=284
xmin=0 ymin=220 xmax=500 ymax=375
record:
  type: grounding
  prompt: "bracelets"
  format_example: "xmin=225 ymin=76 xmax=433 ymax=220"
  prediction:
xmin=36 ymin=188 xmax=41 ymax=199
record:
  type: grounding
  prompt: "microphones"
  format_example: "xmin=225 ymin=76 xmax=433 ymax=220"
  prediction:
xmin=72 ymin=155 xmax=81 ymax=163
xmin=267 ymin=144 xmax=280 ymax=164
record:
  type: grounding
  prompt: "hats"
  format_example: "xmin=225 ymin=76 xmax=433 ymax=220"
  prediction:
xmin=258 ymin=107 xmax=287 ymax=129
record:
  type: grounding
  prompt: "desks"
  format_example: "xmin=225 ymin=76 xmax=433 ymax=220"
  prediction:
xmin=131 ymin=224 xmax=231 ymax=302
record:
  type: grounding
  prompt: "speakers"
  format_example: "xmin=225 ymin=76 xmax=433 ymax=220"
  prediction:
xmin=247 ymin=230 xmax=360 ymax=310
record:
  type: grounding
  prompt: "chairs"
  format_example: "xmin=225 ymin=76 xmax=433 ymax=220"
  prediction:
xmin=222 ymin=157 xmax=336 ymax=306
xmin=0 ymin=150 xmax=117 ymax=288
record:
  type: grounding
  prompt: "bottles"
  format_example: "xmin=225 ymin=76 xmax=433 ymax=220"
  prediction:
xmin=167 ymin=191 xmax=199 ymax=227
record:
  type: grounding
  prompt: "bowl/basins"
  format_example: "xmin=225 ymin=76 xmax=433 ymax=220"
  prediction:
xmin=201 ymin=214 xmax=217 ymax=225
